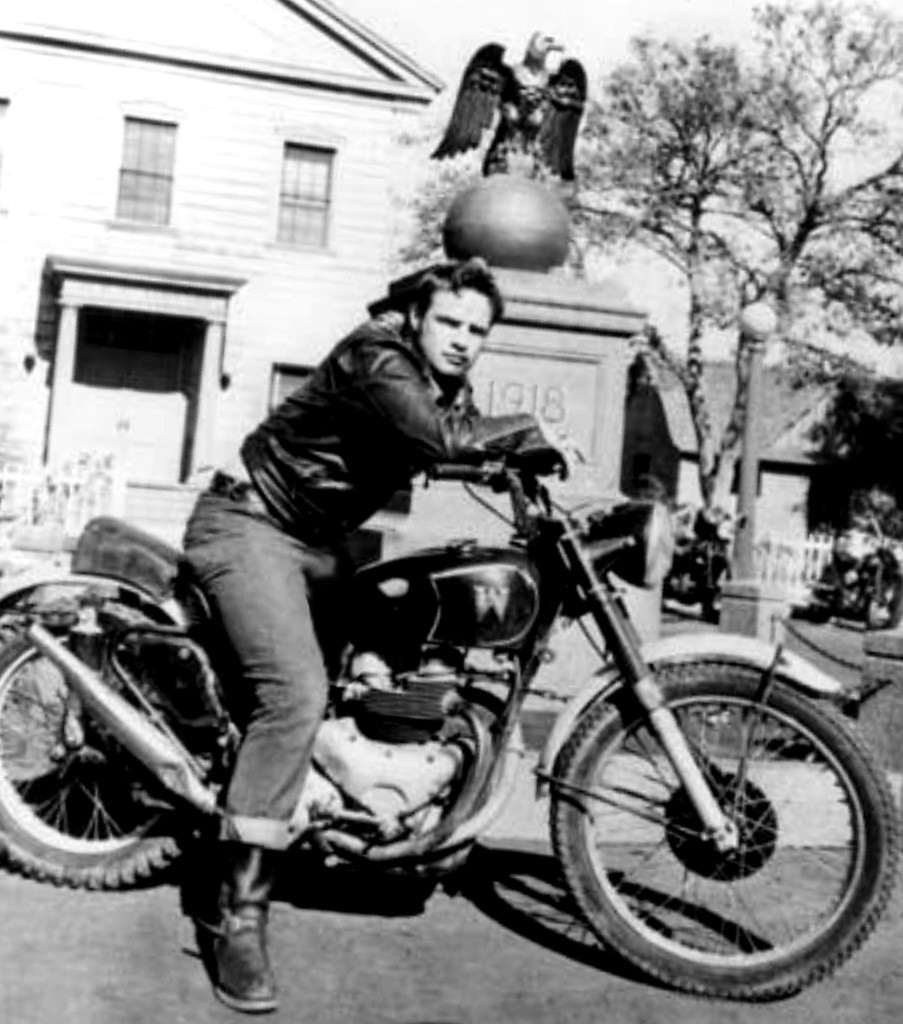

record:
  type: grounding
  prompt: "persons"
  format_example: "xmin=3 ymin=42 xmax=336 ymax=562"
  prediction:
xmin=180 ymin=261 xmax=574 ymax=1015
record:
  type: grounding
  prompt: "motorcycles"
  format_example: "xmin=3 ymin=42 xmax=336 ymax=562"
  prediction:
xmin=1 ymin=444 xmax=901 ymax=1007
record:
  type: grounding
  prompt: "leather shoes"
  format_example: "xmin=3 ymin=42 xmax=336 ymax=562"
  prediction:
xmin=214 ymin=906 xmax=279 ymax=1011
